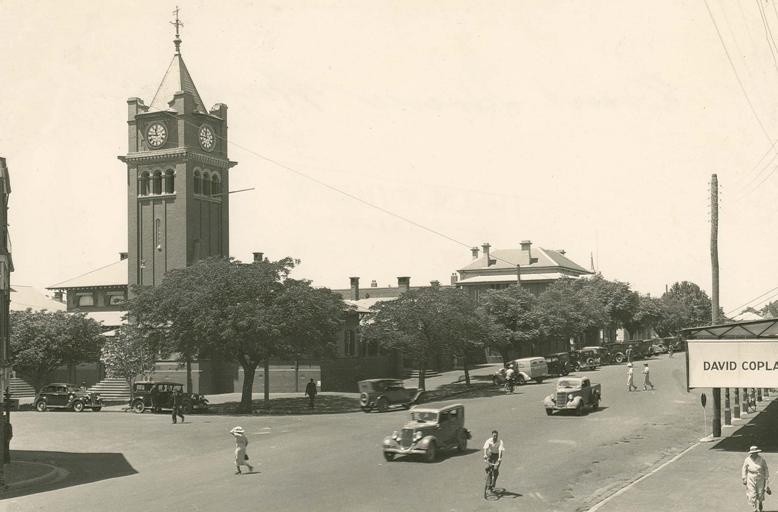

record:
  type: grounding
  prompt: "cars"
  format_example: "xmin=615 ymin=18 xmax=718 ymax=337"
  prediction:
xmin=32 ymin=383 xmax=104 ymax=412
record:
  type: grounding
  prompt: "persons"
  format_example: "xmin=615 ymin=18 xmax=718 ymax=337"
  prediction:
xmin=505 ymin=361 xmax=515 ymax=380
xmin=749 ymin=388 xmax=759 ymax=411
xmin=171 ymin=387 xmax=185 ymax=424
xmin=625 ymin=344 xmax=634 ymax=363
xmin=482 ymin=431 xmax=506 ymax=489
xmin=304 ymin=377 xmax=318 ymax=408
xmin=641 ymin=363 xmax=656 ymax=392
xmin=627 ymin=363 xmax=639 ymax=393
xmin=230 ymin=425 xmax=255 ymax=476
xmin=4 ymin=416 xmax=14 ymax=465
xmin=740 ymin=444 xmax=771 ymax=511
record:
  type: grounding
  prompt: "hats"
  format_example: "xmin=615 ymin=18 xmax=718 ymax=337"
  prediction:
xmin=746 ymin=446 xmax=762 ymax=454
xmin=233 ymin=425 xmax=245 ymax=433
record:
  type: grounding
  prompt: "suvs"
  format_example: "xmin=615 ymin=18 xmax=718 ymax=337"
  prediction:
xmin=128 ymin=381 xmax=210 ymax=413
xmin=356 ymin=378 xmax=424 ymax=413
xmin=382 ymin=401 xmax=472 ymax=463
xmin=494 ymin=335 xmax=682 ymax=384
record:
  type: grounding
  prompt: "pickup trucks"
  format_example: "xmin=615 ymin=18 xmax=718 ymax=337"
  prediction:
xmin=544 ymin=376 xmax=601 ymax=415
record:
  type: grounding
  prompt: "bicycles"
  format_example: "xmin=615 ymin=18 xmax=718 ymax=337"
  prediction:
xmin=502 ymin=373 xmax=520 ymax=395
xmin=484 ymin=458 xmax=500 ymax=498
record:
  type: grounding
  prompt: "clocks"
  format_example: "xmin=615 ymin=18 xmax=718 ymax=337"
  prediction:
xmin=197 ymin=123 xmax=216 ymax=153
xmin=143 ymin=120 xmax=169 ymax=150
